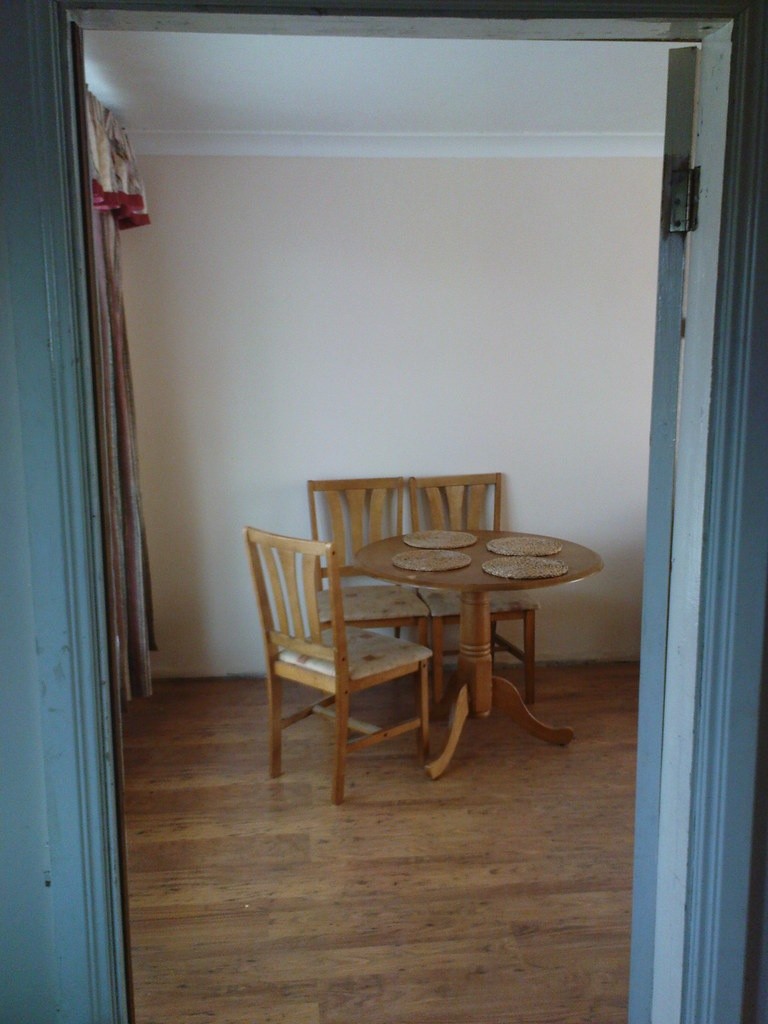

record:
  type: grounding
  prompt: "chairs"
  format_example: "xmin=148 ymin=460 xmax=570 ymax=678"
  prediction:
xmin=406 ymin=473 xmax=537 ymax=704
xmin=305 ymin=476 xmax=430 ymax=647
xmin=244 ymin=526 xmax=432 ymax=805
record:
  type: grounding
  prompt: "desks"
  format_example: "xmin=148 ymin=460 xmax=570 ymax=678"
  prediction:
xmin=357 ymin=529 xmax=604 ymax=777
